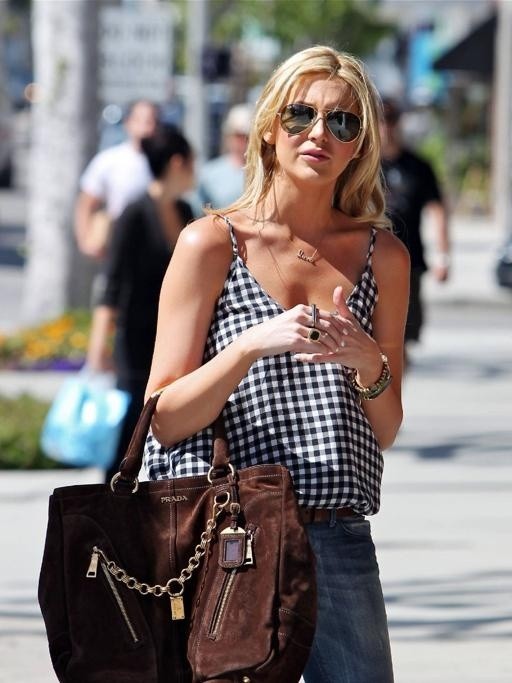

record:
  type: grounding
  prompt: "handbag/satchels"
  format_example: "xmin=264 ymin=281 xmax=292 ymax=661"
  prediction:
xmin=36 ymin=369 xmax=134 ymax=468
xmin=36 ymin=393 xmax=319 ymax=682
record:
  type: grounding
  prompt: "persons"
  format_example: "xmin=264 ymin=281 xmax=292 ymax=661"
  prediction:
xmin=363 ymin=97 xmax=451 ymax=373
xmin=87 ymin=122 xmax=198 ymax=475
xmin=192 ymin=101 xmax=254 ymax=220
xmin=75 ymin=101 xmax=164 ymax=260
xmin=139 ymin=44 xmax=411 ymax=683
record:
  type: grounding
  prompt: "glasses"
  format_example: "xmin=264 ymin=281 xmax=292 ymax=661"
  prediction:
xmin=271 ymin=100 xmax=365 ymax=144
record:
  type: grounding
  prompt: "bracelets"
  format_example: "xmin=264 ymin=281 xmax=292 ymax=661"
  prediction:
xmin=351 ymin=353 xmax=394 ymax=400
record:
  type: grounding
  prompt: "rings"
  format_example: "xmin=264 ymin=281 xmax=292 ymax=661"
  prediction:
xmin=307 ymin=328 xmax=321 ymax=342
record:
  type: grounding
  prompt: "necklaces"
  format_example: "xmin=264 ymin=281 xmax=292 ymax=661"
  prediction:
xmin=263 ymin=196 xmax=333 ymax=264
